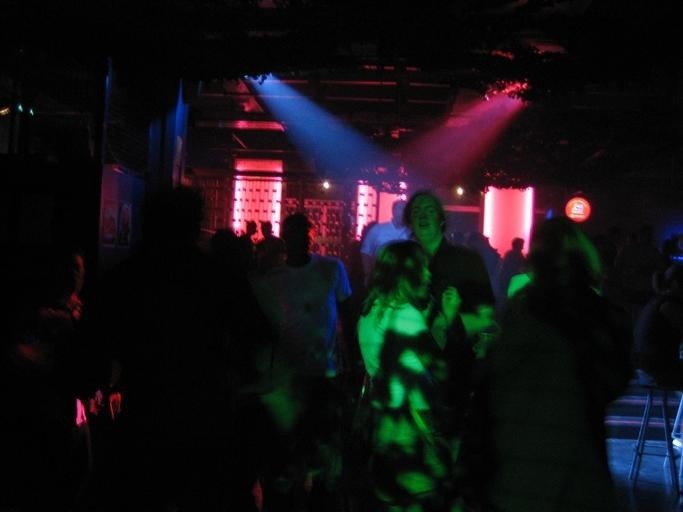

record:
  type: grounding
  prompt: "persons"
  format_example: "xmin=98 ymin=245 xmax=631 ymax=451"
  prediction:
xmin=0 ymin=180 xmax=683 ymax=512
